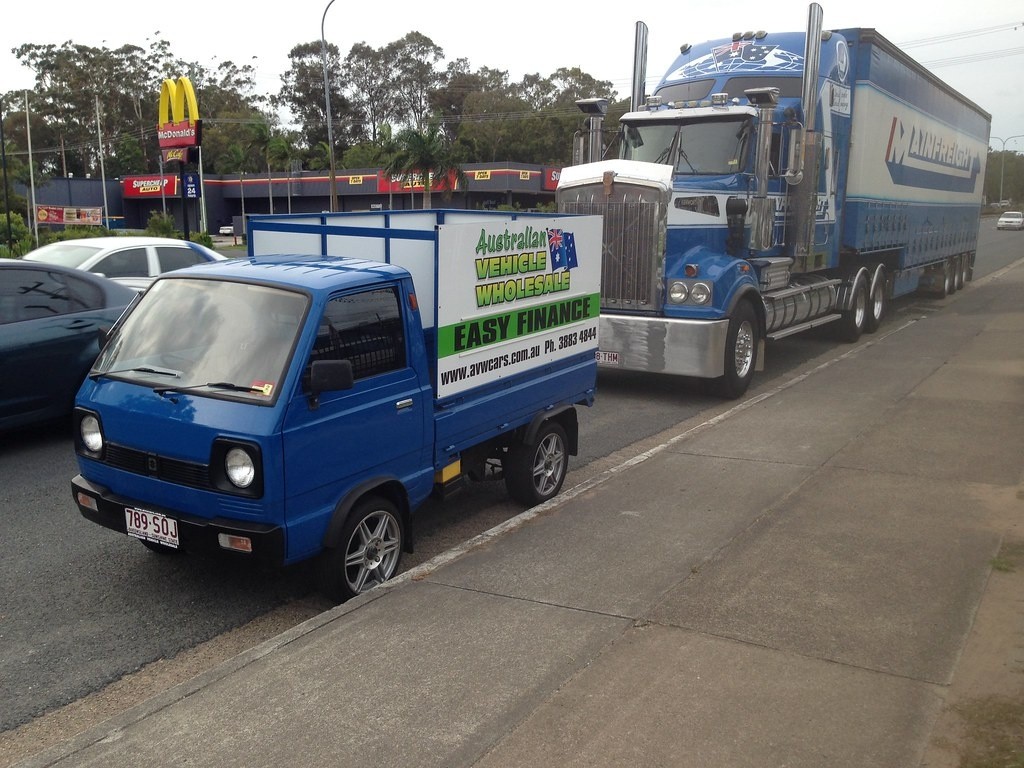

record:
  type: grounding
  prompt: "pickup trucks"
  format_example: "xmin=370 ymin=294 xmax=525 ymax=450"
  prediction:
xmin=217 ymin=222 xmax=234 ymax=236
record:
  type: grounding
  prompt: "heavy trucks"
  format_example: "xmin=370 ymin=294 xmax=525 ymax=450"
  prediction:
xmin=556 ymin=1 xmax=977 ymax=403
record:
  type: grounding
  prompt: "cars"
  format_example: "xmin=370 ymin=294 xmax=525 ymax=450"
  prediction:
xmin=22 ymin=237 xmax=232 ymax=292
xmin=1 ymin=258 xmax=143 ymax=422
xmin=997 ymin=210 xmax=1023 ymax=230
xmin=990 ymin=199 xmax=1011 ymax=210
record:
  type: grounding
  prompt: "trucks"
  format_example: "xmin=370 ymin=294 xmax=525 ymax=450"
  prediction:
xmin=73 ymin=205 xmax=614 ymax=606
